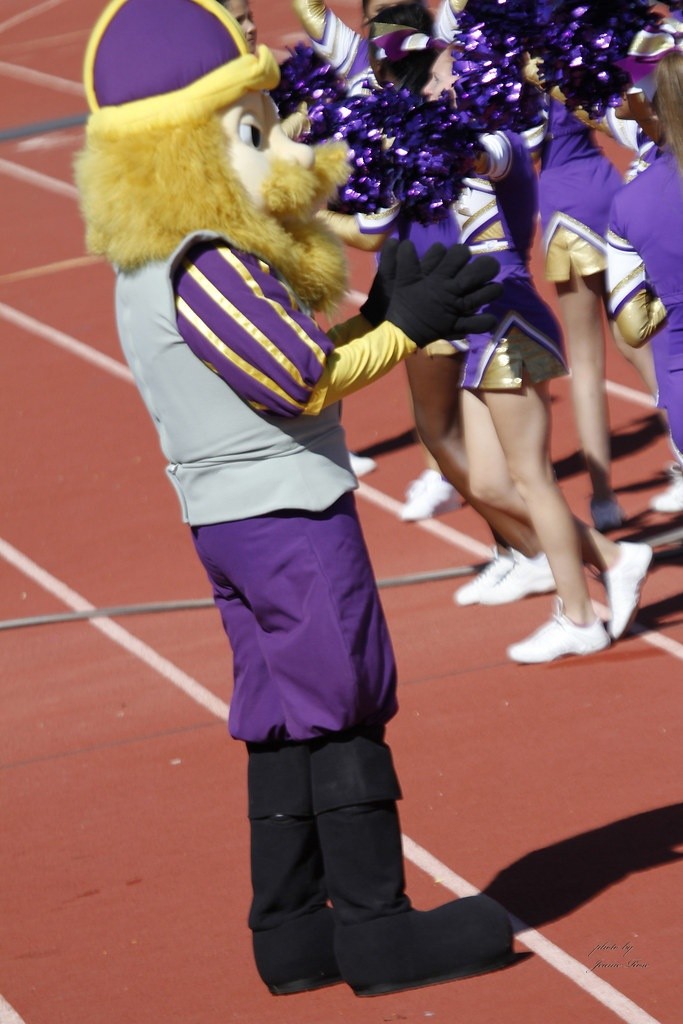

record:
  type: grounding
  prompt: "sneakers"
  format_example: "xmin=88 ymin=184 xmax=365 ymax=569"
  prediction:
xmin=591 ymin=494 xmax=624 ymax=530
xmin=591 ymin=542 xmax=653 ymax=641
xmin=453 ymin=555 xmax=516 ymax=606
xmin=506 ymin=599 xmax=613 ymax=664
xmin=349 ymin=450 xmax=377 ymax=479
xmin=401 ymin=466 xmax=463 ymax=522
xmin=647 ymin=461 xmax=683 ymax=512
xmin=478 ymin=554 xmax=558 ymax=607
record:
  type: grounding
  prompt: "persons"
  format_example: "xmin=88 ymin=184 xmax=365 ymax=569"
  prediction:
xmin=214 ymin=0 xmax=683 ymax=664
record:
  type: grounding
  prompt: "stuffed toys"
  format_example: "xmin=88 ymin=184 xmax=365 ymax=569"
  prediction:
xmin=75 ymin=1 xmax=513 ymax=997
